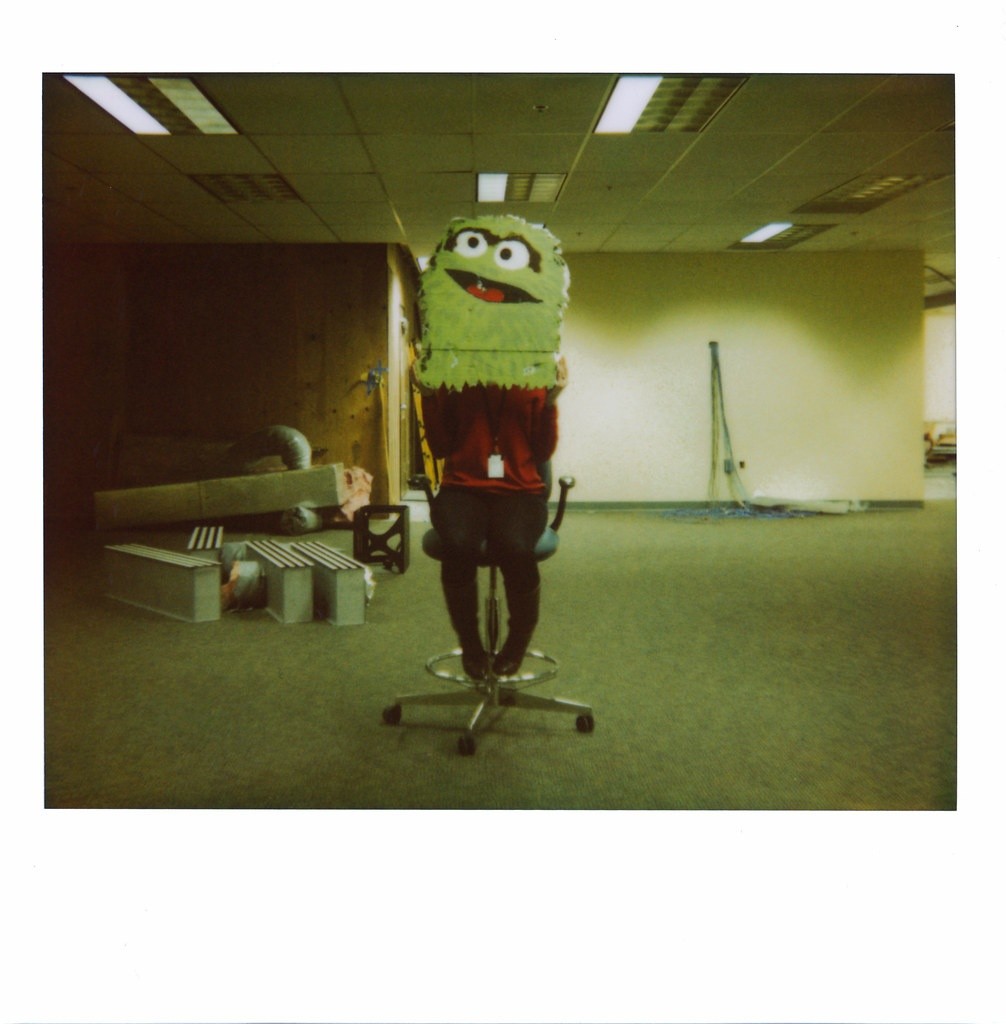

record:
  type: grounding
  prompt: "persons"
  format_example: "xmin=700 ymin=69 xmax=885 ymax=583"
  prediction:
xmin=414 ymin=356 xmax=568 ymax=679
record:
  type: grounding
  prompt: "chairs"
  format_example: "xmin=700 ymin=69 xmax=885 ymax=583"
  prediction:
xmin=383 ymin=474 xmax=594 ymax=756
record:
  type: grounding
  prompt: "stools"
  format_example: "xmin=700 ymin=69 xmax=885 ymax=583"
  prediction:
xmin=354 ymin=504 xmax=410 ymax=574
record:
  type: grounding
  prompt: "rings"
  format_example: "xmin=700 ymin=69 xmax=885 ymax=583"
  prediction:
xmin=562 ymin=376 xmax=566 ymax=380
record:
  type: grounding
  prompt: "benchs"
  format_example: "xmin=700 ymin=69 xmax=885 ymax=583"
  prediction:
xmin=102 ymin=526 xmax=365 ymax=626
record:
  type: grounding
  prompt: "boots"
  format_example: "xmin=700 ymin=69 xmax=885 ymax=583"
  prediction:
xmin=493 ymin=585 xmax=540 ymax=673
xmin=444 ymin=586 xmax=488 ymax=680
